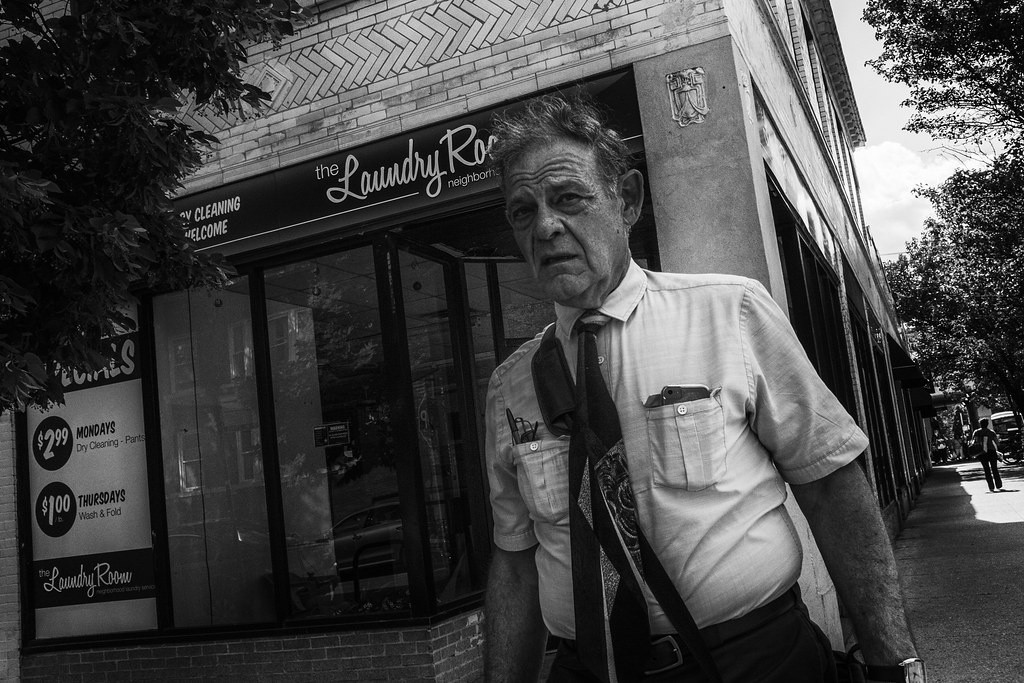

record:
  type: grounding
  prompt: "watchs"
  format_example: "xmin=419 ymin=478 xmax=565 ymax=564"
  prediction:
xmin=868 ymin=657 xmax=927 ymax=683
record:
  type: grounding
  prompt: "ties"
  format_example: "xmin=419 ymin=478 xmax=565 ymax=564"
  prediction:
xmin=569 ymin=309 xmax=651 ymax=683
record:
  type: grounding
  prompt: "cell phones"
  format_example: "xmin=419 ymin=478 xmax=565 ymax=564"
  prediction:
xmin=662 ymin=385 xmax=710 ymax=407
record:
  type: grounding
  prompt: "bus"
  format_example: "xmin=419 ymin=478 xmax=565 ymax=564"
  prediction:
xmin=990 ymin=410 xmax=1024 ymax=443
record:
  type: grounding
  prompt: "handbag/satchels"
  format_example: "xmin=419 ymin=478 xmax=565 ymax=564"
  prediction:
xmin=967 ymin=430 xmax=987 ymax=459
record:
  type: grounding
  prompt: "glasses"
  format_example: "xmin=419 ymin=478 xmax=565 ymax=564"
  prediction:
xmin=512 ymin=417 xmax=539 ymax=446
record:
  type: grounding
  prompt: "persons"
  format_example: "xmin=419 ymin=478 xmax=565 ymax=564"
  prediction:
xmin=972 ymin=418 xmax=1002 ymax=491
xmin=930 ymin=430 xmax=968 ymax=465
xmin=482 ymin=95 xmax=927 ymax=683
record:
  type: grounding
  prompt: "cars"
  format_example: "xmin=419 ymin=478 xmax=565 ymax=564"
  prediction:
xmin=169 ymin=498 xmax=406 ymax=604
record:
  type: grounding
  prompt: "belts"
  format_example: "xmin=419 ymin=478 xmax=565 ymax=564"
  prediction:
xmin=562 ymin=586 xmax=801 ymax=677
